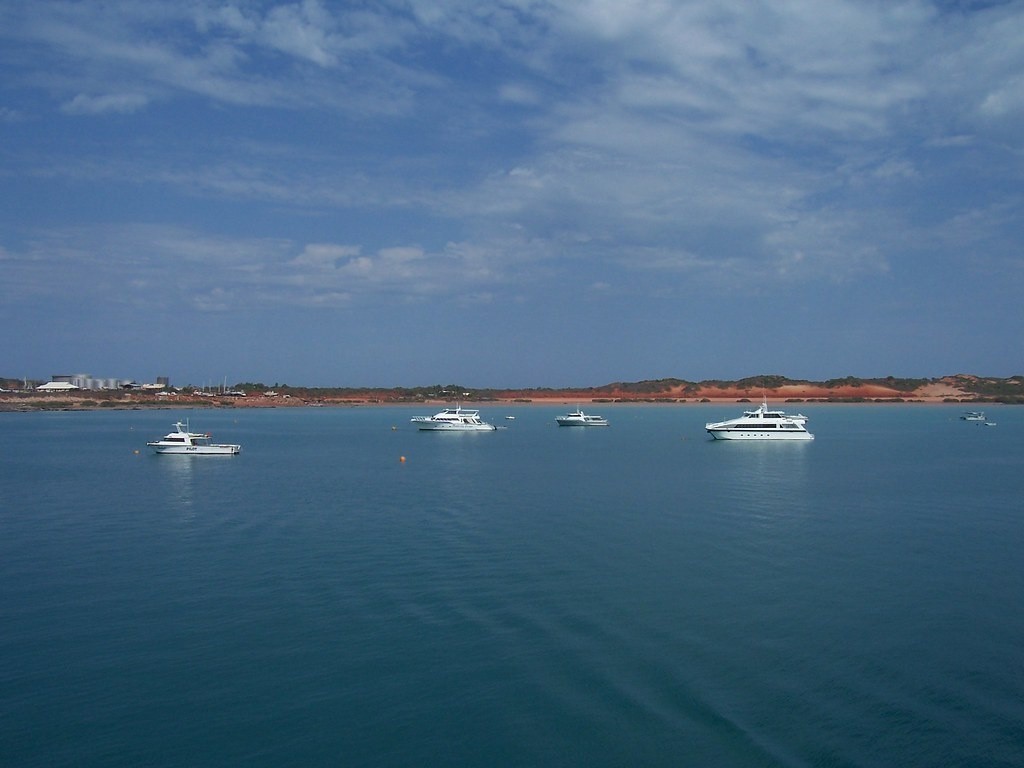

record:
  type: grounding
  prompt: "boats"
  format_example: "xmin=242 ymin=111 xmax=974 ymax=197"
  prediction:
xmin=959 ymin=410 xmax=997 ymax=426
xmin=409 ymin=401 xmax=500 ymax=431
xmin=704 ymin=383 xmax=816 ymax=441
xmin=555 ymin=403 xmax=610 ymax=426
xmin=505 ymin=415 xmax=516 ymax=420
xmin=146 ymin=418 xmax=242 ymax=454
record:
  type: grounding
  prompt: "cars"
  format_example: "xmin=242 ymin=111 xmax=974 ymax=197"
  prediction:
xmin=154 ymin=391 xmax=216 ymax=397
xmin=262 ymin=391 xmax=278 ymax=397
